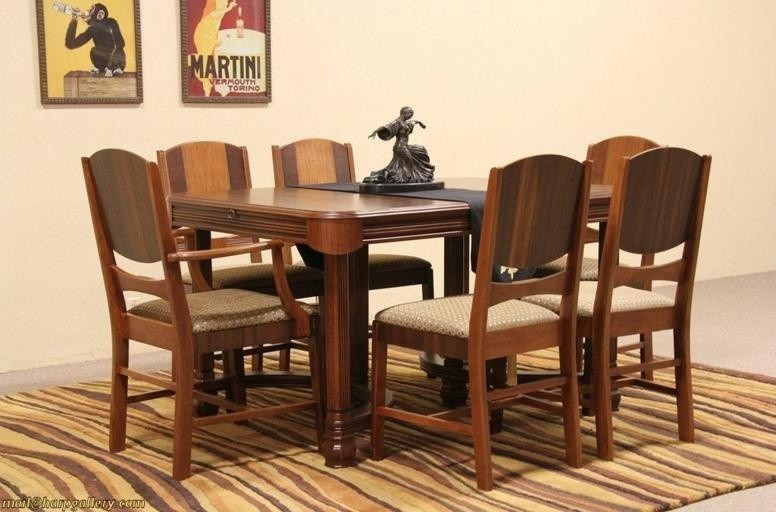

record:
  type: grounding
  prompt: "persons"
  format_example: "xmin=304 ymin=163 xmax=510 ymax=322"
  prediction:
xmin=365 ymin=105 xmax=435 ymax=182
xmin=187 ymin=0 xmax=237 ymax=98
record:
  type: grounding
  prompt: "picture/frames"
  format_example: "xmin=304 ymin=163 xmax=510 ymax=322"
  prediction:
xmin=35 ymin=0 xmax=144 ymax=106
xmin=179 ymin=0 xmax=273 ymax=104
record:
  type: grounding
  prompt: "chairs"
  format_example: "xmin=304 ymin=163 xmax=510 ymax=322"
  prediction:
xmin=81 ymin=137 xmax=712 ymax=493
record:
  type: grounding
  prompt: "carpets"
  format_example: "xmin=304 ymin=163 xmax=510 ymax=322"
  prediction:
xmin=0 ymin=336 xmax=776 ymax=512
